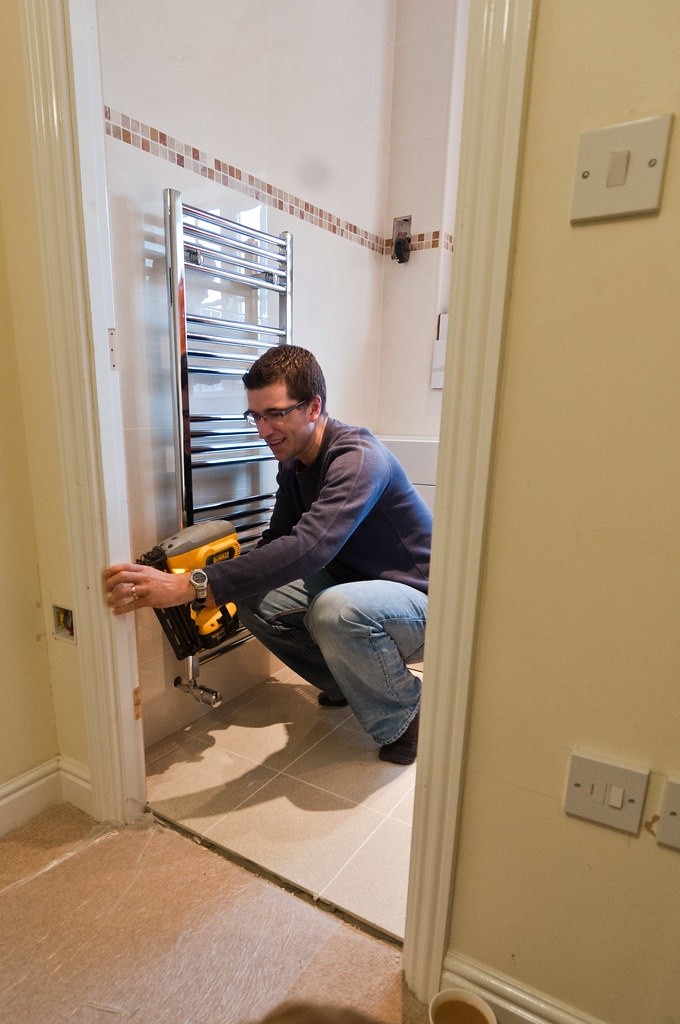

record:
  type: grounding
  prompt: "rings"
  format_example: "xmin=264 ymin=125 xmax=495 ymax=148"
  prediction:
xmin=131 ymin=585 xmax=139 ymax=601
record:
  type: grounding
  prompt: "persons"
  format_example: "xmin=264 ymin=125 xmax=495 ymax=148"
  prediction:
xmin=102 ymin=344 xmax=432 ymax=766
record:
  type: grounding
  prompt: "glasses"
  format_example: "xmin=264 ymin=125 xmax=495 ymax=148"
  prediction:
xmin=244 ymin=401 xmax=305 ymax=426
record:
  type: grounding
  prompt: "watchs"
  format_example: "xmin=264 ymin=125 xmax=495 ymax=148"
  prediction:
xmin=189 ymin=568 xmax=208 ymax=609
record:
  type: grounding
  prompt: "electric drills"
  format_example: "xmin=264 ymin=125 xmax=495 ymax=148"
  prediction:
xmin=132 ymin=519 xmax=242 ymax=661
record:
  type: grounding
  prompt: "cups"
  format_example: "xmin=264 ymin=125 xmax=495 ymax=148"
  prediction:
xmin=428 ymin=987 xmax=497 ymax=1024
